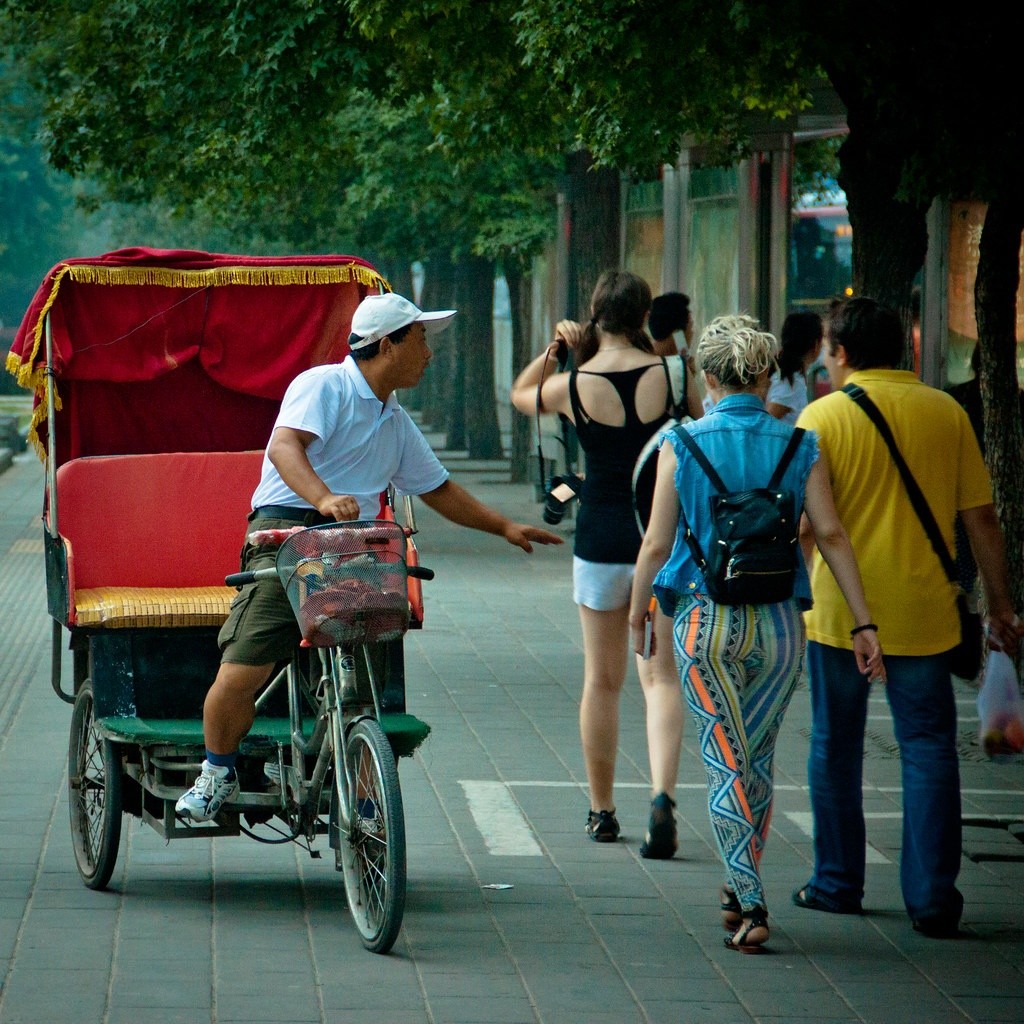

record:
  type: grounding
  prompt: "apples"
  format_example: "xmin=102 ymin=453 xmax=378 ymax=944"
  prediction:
xmin=983 ymin=710 xmax=1024 ymax=756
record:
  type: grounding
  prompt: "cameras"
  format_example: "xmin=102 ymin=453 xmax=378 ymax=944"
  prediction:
xmin=543 ymin=473 xmax=584 ymax=525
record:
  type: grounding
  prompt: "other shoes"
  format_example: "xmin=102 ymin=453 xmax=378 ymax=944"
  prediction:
xmin=639 ymin=793 xmax=677 ymax=859
xmin=911 ymin=921 xmax=950 ymax=940
xmin=585 ymin=809 xmax=618 ymax=841
xmin=792 ymin=886 xmax=863 ymax=914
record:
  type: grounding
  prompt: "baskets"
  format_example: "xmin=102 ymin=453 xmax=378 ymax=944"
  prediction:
xmin=276 ymin=522 xmax=411 ymax=647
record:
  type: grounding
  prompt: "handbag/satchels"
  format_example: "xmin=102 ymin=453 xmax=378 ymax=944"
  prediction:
xmin=977 ymin=649 xmax=1024 ymax=756
xmin=950 ymin=588 xmax=984 ymax=680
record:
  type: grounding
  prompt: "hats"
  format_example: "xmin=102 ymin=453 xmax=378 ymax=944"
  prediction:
xmin=347 ymin=293 xmax=456 ymax=350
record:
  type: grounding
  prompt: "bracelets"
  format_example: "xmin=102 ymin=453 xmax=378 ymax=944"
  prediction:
xmin=850 ymin=624 xmax=878 ymax=640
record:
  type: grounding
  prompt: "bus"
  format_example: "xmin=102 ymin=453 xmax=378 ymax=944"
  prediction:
xmin=619 ymin=193 xmax=920 ymax=410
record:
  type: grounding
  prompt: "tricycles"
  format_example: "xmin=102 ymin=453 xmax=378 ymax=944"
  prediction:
xmin=40 ymin=249 xmax=436 ymax=955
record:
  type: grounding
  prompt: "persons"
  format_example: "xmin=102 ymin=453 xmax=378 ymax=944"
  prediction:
xmin=174 ymin=292 xmax=567 ymax=822
xmin=509 ymin=268 xmax=1024 ymax=955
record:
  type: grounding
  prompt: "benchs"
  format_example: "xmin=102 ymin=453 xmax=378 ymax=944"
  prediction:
xmin=42 ymin=449 xmax=422 ymax=632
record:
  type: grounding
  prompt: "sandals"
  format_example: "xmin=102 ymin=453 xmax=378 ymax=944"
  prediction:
xmin=719 ymin=887 xmax=743 ymax=931
xmin=724 ymin=906 xmax=768 ymax=954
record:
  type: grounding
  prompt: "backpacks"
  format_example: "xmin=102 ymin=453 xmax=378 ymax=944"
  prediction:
xmin=669 ymin=423 xmax=804 ymax=607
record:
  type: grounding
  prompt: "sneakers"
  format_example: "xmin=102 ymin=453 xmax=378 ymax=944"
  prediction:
xmin=355 ymin=809 xmax=383 ymax=859
xmin=176 ymin=759 xmax=242 ymax=823
